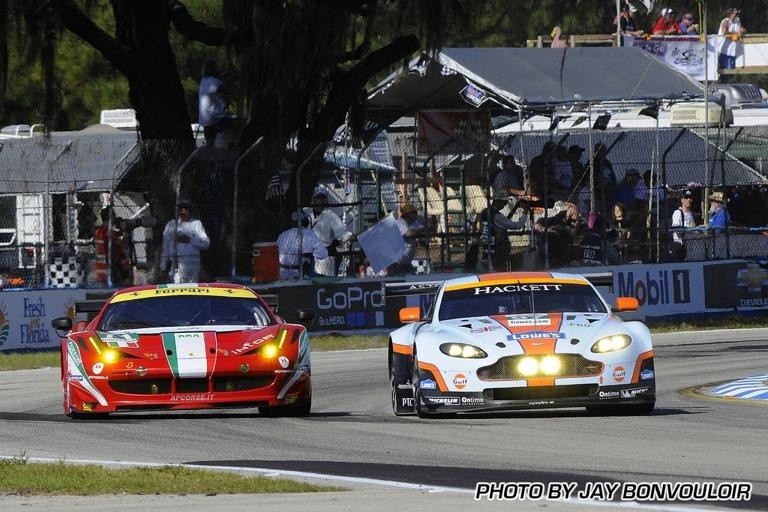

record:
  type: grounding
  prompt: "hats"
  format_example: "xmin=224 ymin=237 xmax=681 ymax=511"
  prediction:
xmin=490 ymin=189 xmax=511 ymax=201
xmin=661 ymin=8 xmax=674 ymax=18
xmin=400 ymin=203 xmax=417 ymax=218
xmin=680 ymin=189 xmax=694 ymax=199
xmin=292 ymin=208 xmax=311 ymax=222
xmin=706 ymin=191 xmax=731 ymax=203
xmin=313 ymin=187 xmax=327 ymax=198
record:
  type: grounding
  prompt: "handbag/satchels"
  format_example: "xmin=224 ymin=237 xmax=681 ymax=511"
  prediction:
xmin=666 ymin=239 xmax=687 ymax=262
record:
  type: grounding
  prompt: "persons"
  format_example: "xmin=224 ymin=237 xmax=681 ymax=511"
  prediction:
xmin=615 ymin=0 xmax=702 ymax=36
xmin=387 ymin=204 xmax=426 ymax=274
xmin=95 ymin=208 xmax=129 ymax=284
xmin=476 ymin=141 xmax=730 ymax=270
xmin=551 ymin=18 xmax=570 ymax=47
xmin=716 ymin=9 xmax=748 ymax=79
xmin=303 ymin=187 xmax=355 ymax=276
xmin=159 ymin=200 xmax=210 ymax=282
xmin=198 ymin=59 xmax=240 ymax=144
xmin=276 ymin=212 xmax=328 ymax=280
xmin=109 ymin=208 xmax=157 ymax=286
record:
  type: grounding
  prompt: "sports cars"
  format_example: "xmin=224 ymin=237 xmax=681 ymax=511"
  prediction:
xmin=386 ymin=270 xmax=657 ymax=415
xmin=51 ymin=281 xmax=312 ymax=419
